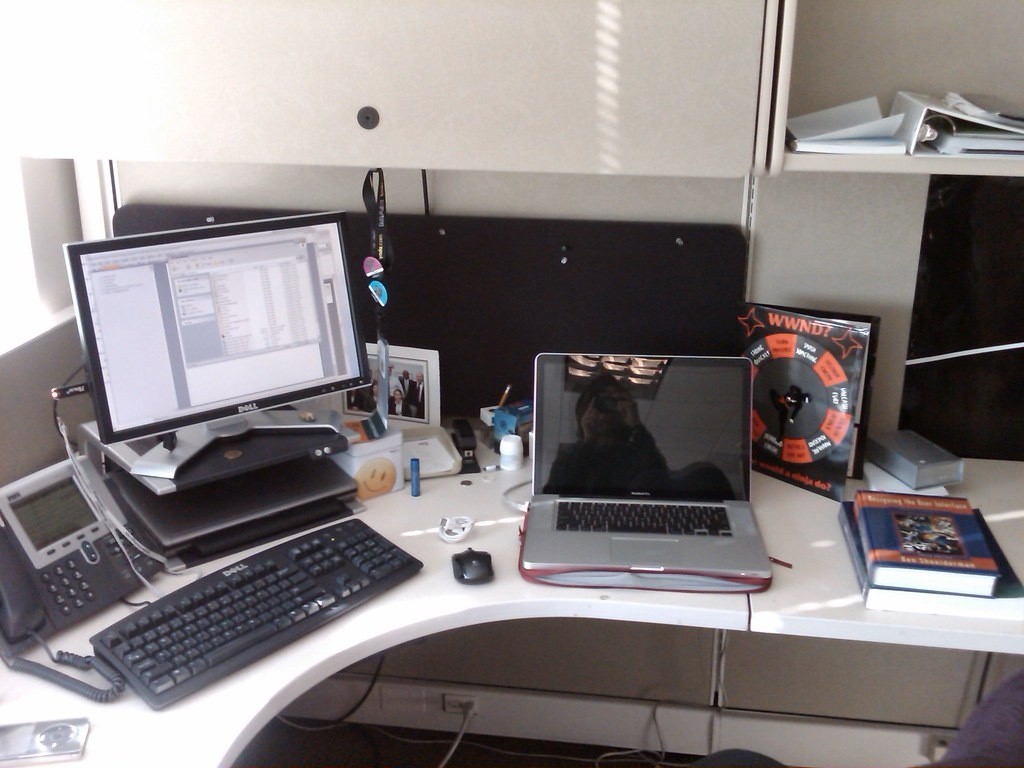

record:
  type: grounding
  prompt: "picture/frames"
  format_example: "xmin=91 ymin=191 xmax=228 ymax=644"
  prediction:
xmin=335 ymin=343 xmax=442 ymax=430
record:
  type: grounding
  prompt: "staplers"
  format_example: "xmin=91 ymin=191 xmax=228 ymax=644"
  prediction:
xmin=451 ymin=417 xmax=481 ymax=474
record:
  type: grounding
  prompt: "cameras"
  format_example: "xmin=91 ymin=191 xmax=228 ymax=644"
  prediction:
xmin=595 ymin=396 xmax=620 ymax=415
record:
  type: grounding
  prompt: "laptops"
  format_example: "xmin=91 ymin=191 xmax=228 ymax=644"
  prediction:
xmin=522 ymin=353 xmax=773 ymax=579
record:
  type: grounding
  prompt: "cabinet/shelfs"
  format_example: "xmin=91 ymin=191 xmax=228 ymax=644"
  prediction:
xmin=0 ymin=0 xmax=1024 ymax=451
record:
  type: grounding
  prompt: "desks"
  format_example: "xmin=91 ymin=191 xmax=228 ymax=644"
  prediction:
xmin=0 ymin=304 xmax=1024 ymax=768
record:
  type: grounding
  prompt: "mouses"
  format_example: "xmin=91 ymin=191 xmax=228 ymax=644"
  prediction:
xmin=451 ymin=547 xmax=494 ymax=585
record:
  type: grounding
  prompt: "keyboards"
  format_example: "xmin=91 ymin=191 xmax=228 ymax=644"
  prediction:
xmin=88 ymin=518 xmax=423 ymax=709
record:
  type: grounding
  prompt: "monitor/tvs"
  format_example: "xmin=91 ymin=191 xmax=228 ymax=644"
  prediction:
xmin=63 ymin=209 xmax=372 ymax=480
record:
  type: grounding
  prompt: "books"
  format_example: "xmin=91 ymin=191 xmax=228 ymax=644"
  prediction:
xmin=785 ymin=94 xmax=910 ymax=155
xmin=837 ymin=488 xmax=1024 ymax=621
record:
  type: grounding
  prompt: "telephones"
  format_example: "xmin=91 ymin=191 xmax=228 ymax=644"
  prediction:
xmin=0 ymin=454 xmax=166 ymax=659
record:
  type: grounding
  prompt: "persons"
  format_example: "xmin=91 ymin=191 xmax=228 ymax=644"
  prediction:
xmin=679 ymin=664 xmax=1024 ymax=768
xmin=542 ymin=375 xmax=733 ymax=502
xmin=346 ymin=363 xmax=427 ymax=419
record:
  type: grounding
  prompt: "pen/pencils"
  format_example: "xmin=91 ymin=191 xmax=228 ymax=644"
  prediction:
xmin=496 ymin=385 xmax=512 ymax=408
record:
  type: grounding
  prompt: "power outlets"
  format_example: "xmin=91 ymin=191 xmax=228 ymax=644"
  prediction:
xmin=443 ymin=694 xmax=480 ymax=714
